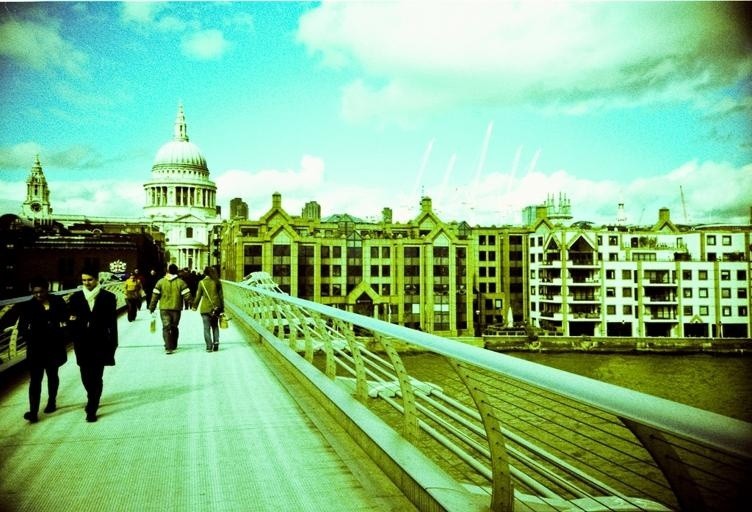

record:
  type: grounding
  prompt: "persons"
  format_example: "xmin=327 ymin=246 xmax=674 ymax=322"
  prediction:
xmin=191 ymin=266 xmax=225 ymax=352
xmin=16 ymin=276 xmax=72 ymax=423
xmin=172 ymin=265 xmax=207 ymax=310
xmin=148 ymin=263 xmax=194 ymax=353
xmin=63 ymin=265 xmax=119 ymax=423
xmin=122 ymin=272 xmax=147 ymax=322
xmin=142 ymin=269 xmax=163 ymax=309
xmin=132 ymin=268 xmax=146 ymax=311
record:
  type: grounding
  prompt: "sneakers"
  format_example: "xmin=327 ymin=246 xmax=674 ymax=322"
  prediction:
xmin=165 ymin=346 xmax=176 ymax=354
xmin=44 ymin=403 xmax=56 ymax=414
xmin=24 ymin=412 xmax=38 ymax=423
xmin=213 ymin=342 xmax=218 ymax=351
xmin=206 ymin=343 xmax=213 ymax=352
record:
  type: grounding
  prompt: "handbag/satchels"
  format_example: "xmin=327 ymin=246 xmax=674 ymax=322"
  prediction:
xmin=127 ymin=290 xmax=138 ymax=299
xmin=219 ymin=314 xmax=228 ymax=328
xmin=149 ymin=319 xmax=156 ymax=334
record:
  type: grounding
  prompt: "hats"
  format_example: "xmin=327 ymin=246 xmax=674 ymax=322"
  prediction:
xmin=169 ymin=264 xmax=178 ymax=272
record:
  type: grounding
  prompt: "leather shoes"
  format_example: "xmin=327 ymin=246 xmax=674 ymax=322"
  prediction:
xmin=85 ymin=402 xmax=97 ymax=420
